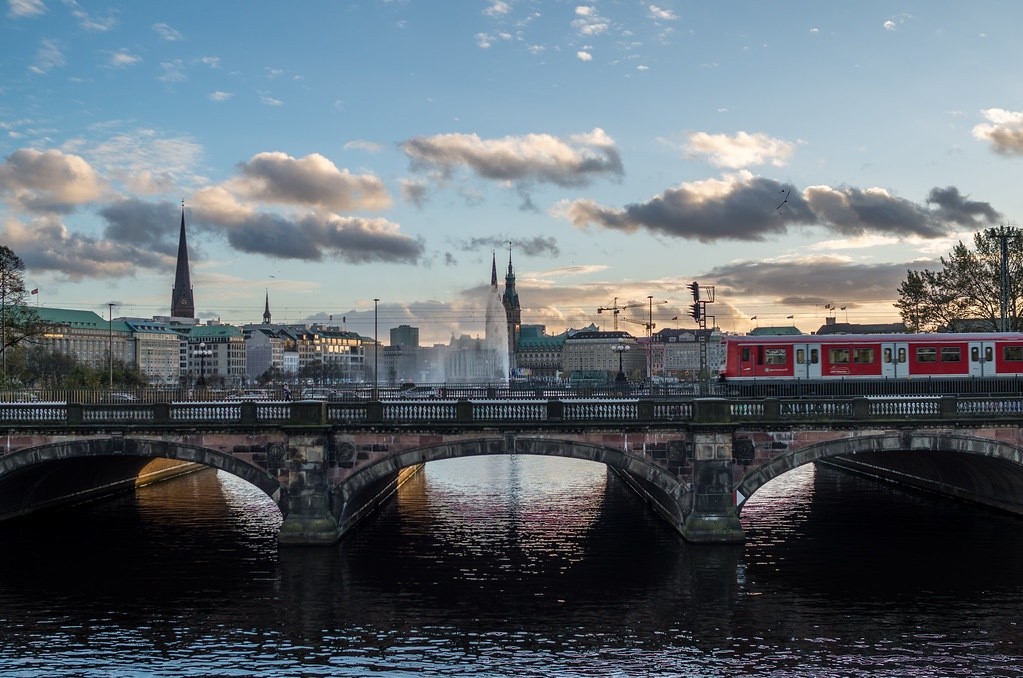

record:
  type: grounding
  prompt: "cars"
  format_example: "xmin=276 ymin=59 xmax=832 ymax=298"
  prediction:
xmin=102 ymin=392 xmax=139 ymax=407
xmin=1 ymin=390 xmax=42 ymax=409
xmin=399 ymin=385 xmax=442 ymax=402
xmin=334 ymin=390 xmax=363 ymax=404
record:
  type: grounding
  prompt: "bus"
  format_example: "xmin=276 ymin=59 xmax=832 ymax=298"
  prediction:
xmin=717 ymin=326 xmax=1023 ymax=403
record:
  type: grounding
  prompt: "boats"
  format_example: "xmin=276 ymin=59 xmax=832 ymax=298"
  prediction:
xmin=224 ymin=390 xmax=270 ymax=406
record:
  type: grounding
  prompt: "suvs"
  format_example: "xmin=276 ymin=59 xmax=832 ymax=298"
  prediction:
xmin=300 ymin=387 xmax=340 ymax=405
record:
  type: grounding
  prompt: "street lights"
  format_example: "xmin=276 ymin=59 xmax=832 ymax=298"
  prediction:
xmin=192 ymin=342 xmax=212 ymax=403
xmin=610 ymin=338 xmax=631 ymax=396
